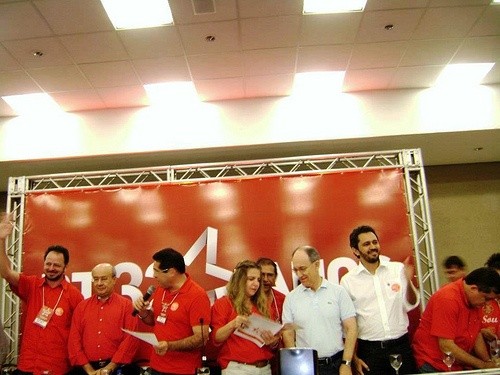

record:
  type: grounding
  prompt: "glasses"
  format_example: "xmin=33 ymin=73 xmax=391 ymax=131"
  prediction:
xmin=292 ymin=259 xmax=318 ymax=273
xmin=154 ymin=267 xmax=173 ymax=274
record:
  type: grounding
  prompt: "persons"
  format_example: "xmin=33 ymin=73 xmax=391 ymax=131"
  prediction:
xmin=340 ymin=226 xmax=420 ymax=375
xmin=465 ymin=253 xmax=500 ymax=370
xmin=442 ymin=255 xmax=467 ymax=282
xmin=282 ymin=246 xmax=358 ymax=375
xmin=68 ymin=263 xmax=140 ymax=375
xmin=133 ymin=248 xmax=212 ymax=375
xmin=0 ymin=211 xmax=84 ymax=375
xmin=257 ymin=258 xmax=285 ymax=375
xmin=209 ymin=261 xmax=280 ymax=375
xmin=412 ymin=267 xmax=500 ymax=373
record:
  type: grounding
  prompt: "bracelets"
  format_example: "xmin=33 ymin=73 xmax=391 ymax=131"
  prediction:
xmin=139 ymin=310 xmax=152 ymax=322
xmin=341 ymin=360 xmax=351 ymax=366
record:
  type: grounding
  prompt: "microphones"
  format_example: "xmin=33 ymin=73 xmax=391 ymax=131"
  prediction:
xmin=200 ymin=317 xmax=208 ymax=374
xmin=131 ymin=285 xmax=156 ymax=317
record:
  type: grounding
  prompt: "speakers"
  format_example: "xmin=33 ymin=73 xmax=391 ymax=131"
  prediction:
xmin=279 ymin=347 xmax=317 ymax=375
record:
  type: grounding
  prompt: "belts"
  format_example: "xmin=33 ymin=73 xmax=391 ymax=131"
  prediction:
xmin=94 ymin=360 xmax=109 ymax=367
xmin=235 ymin=361 xmax=268 ymax=367
xmin=319 ymin=352 xmax=344 ymax=365
xmin=358 ymin=336 xmax=407 ymax=348
